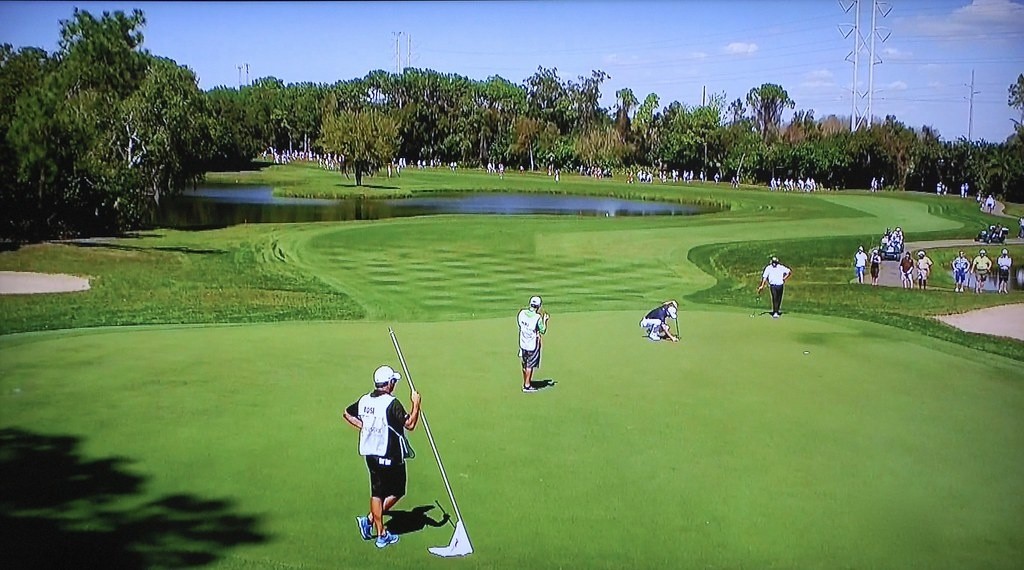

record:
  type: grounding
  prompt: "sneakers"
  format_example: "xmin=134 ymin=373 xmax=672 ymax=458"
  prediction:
xmin=375 ymin=530 xmax=398 ymax=547
xmin=357 ymin=515 xmax=374 ymax=540
xmin=645 ymin=329 xmax=650 ymax=336
xmin=523 ymin=386 xmax=539 ymax=392
xmin=649 ymin=333 xmax=660 ymax=340
xmin=773 ymin=312 xmax=779 ymax=318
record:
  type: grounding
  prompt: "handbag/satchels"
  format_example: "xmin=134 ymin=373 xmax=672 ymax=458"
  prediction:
xmin=398 ymin=434 xmax=417 ymax=463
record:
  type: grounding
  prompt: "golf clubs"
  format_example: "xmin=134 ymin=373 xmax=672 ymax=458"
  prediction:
xmin=675 ymin=304 xmax=680 ymax=338
xmin=749 ymin=289 xmax=761 ymax=318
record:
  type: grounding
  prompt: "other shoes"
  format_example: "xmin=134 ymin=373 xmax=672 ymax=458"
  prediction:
xmin=954 ymin=287 xmax=959 ymax=292
xmin=960 ymin=288 xmax=964 ymax=292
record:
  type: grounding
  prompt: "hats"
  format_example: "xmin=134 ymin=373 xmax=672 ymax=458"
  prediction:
xmin=859 ymin=245 xmax=864 ymax=251
xmin=917 ymin=250 xmax=925 ymax=256
xmin=668 ymin=307 xmax=677 ymax=319
xmin=374 ymin=365 xmax=401 ymax=383
xmin=1002 ymin=248 xmax=1008 ymax=255
xmin=530 ymin=296 xmax=542 ymax=306
xmin=873 ymin=250 xmax=878 ymax=254
xmin=979 ymin=249 xmax=986 ymax=254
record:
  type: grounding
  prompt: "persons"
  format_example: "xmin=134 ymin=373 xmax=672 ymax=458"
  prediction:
xmin=916 ymin=251 xmax=932 ymax=289
xmin=517 ymin=296 xmax=549 ymax=392
xmin=889 ymin=227 xmax=903 ymax=252
xmin=952 ymin=252 xmax=970 ymax=292
xmin=937 ymin=182 xmax=948 ymax=195
xmin=994 ymin=223 xmax=1005 ymax=245
xmin=900 ymin=252 xmax=914 ymax=288
xmin=344 ymin=364 xmax=421 ymax=548
xmin=640 ymin=301 xmax=679 ymax=341
xmin=771 ymin=177 xmax=816 ymax=192
xmin=871 ymin=177 xmax=878 ymax=192
xmin=1018 ymin=215 xmax=1024 ymax=239
xmin=263 ymin=147 xmax=739 ymax=189
xmin=976 ymin=190 xmax=994 ymax=213
xmin=758 ymin=256 xmax=791 ymax=318
xmin=870 ymin=250 xmax=881 ymax=285
xmin=997 ymin=249 xmax=1011 ymax=294
xmin=970 ymin=249 xmax=992 ymax=293
xmin=960 ymin=183 xmax=969 ymax=198
xmin=854 ymin=245 xmax=868 ymax=284
